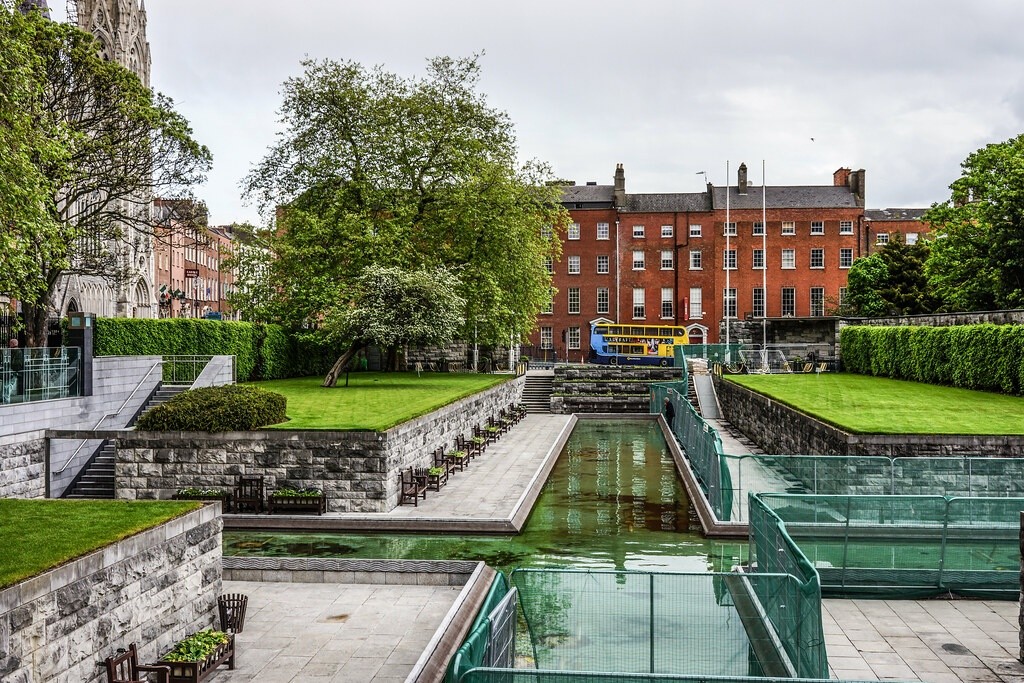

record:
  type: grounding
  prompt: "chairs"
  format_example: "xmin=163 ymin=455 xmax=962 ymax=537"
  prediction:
xmin=105 ymin=642 xmax=171 ymax=683
xmin=434 ymin=446 xmax=455 ymax=481
xmin=400 ymin=466 xmax=428 ymax=507
xmin=510 ymin=403 xmax=524 ymax=421
xmin=456 ymin=433 xmax=475 ymax=463
xmin=233 ymin=475 xmax=264 ymax=515
xmin=500 ymin=408 xmax=517 ymax=428
xmin=474 ymin=423 xmax=490 ymax=449
xmin=489 ymin=414 xmax=503 ymax=438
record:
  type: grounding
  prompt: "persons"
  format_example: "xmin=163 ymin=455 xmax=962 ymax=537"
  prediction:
xmin=2 ymin=338 xmax=25 ymax=397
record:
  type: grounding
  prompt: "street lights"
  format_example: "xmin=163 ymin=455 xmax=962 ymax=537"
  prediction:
xmin=696 ymin=170 xmax=706 ymax=182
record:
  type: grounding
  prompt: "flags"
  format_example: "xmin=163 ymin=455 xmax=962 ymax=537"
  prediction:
xmin=206 ymin=287 xmax=210 ymax=295
xmin=158 ymin=285 xmax=182 ymax=301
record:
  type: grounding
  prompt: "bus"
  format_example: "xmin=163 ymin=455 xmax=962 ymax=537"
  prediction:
xmin=590 ymin=325 xmax=689 ymax=366
